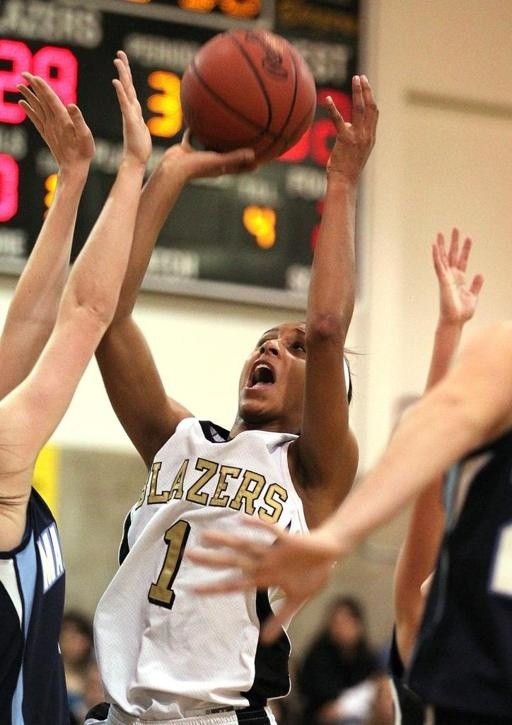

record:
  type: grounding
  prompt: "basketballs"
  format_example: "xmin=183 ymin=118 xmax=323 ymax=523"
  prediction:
xmin=181 ymin=25 xmax=318 ymax=165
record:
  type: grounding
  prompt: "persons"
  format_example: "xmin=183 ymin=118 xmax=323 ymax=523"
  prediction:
xmin=0 ymin=48 xmax=512 ymax=724
